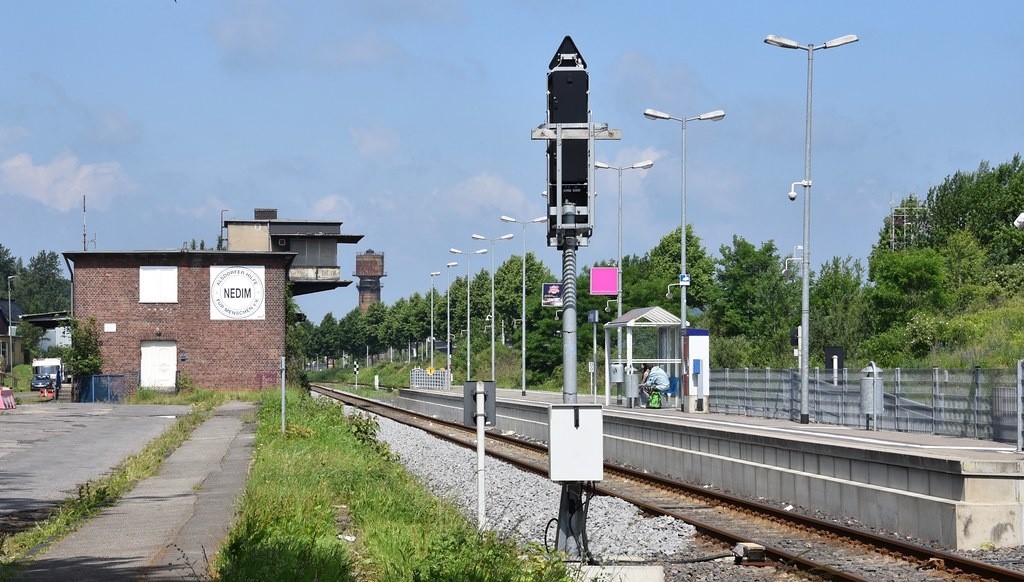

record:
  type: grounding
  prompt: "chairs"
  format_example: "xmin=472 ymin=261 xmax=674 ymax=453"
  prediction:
xmin=37 ymin=377 xmax=40 ymax=380
xmin=45 ymin=377 xmax=48 ymax=379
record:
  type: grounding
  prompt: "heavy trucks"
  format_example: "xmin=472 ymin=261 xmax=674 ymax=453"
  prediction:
xmin=32 ymin=357 xmax=64 ymax=389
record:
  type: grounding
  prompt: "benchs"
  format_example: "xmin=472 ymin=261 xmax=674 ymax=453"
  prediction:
xmin=655 ymin=377 xmax=681 ymax=407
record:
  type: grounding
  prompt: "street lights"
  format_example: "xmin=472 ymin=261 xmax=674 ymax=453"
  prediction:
xmin=447 ymin=262 xmax=458 ymax=386
xmin=763 ymin=33 xmax=858 ymax=425
xmin=449 ymin=247 xmax=487 ymax=379
xmin=591 ymin=160 xmax=655 ymax=405
xmin=471 ymin=234 xmax=515 ymax=380
xmin=500 ymin=214 xmax=548 ymax=396
xmin=7 ymin=276 xmax=19 ymax=385
xmin=643 ymin=108 xmax=725 ymax=326
xmin=430 ymin=272 xmax=440 ymax=369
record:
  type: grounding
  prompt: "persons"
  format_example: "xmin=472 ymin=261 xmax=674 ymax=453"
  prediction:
xmin=639 ymin=363 xmax=670 ymax=408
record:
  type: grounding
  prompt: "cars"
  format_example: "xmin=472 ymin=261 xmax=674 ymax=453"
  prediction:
xmin=29 ymin=374 xmax=53 ymax=392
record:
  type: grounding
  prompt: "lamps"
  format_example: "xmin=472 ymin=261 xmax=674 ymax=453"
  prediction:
xmin=155 ymin=327 xmax=161 ymax=336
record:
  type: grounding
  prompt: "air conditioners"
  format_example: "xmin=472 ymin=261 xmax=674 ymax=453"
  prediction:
xmin=279 ymin=239 xmax=286 ymax=246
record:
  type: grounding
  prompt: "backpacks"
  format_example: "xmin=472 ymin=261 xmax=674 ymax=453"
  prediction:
xmin=646 ymin=392 xmax=662 ymax=408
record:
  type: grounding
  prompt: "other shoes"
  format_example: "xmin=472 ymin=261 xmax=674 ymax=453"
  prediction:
xmin=640 ymin=404 xmax=650 ymax=408
